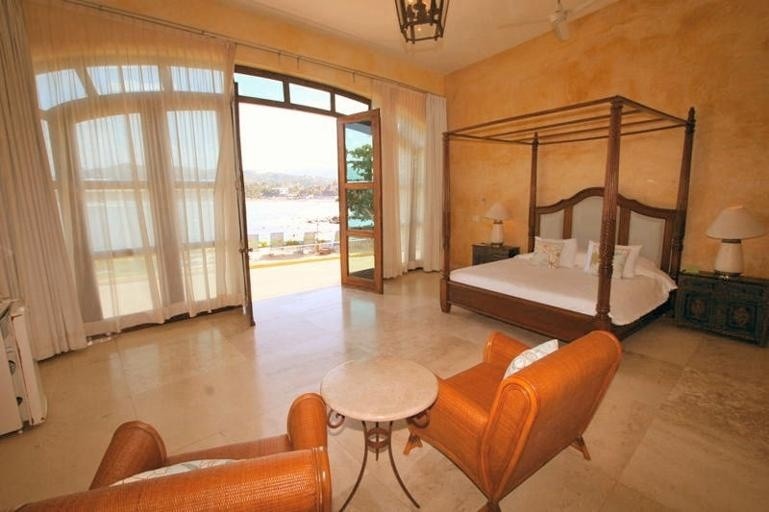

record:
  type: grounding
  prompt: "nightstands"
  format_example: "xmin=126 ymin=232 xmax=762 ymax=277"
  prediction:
xmin=674 ymin=269 xmax=768 ymax=347
xmin=470 ymin=242 xmax=519 ymax=266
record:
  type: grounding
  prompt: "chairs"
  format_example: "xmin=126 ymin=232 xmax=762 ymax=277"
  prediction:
xmin=6 ymin=395 xmax=331 ymax=511
xmin=247 ymin=217 xmax=342 ymax=260
xmin=413 ymin=327 xmax=622 ymax=511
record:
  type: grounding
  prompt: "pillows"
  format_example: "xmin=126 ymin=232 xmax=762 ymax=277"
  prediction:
xmin=587 ymin=238 xmax=628 ymax=279
xmin=109 ymin=456 xmax=239 ymax=487
xmin=596 ymin=241 xmax=642 ymax=279
xmin=534 ymin=235 xmax=577 ymax=270
xmin=501 ymin=336 xmax=558 ymax=381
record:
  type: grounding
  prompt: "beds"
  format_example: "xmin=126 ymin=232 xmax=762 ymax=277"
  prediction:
xmin=441 ymin=94 xmax=697 ymax=343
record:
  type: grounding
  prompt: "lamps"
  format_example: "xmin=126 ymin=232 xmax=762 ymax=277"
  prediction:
xmin=485 ymin=201 xmax=511 ymax=248
xmin=395 ymin=1 xmax=450 ymax=45
xmin=705 ymin=202 xmax=768 ymax=277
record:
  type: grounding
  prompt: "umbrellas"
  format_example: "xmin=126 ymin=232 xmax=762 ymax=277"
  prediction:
xmin=304 ymin=215 xmax=330 ymax=238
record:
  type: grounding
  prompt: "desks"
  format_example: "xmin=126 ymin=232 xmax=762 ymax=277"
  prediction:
xmin=321 ymin=357 xmax=438 ymax=511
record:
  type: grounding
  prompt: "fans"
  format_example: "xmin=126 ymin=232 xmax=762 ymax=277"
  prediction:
xmin=495 ymin=0 xmax=608 ymax=41
